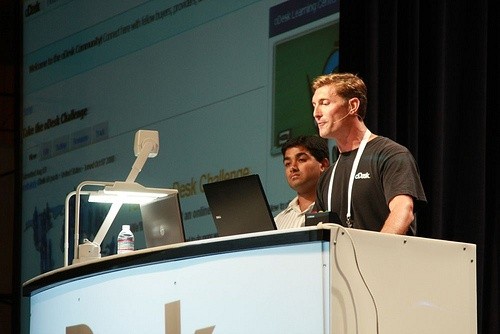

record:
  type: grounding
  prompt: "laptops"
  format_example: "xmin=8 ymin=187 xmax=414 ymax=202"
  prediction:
xmin=140 ymin=194 xmax=187 ymax=248
xmin=203 ymin=174 xmax=278 ymax=236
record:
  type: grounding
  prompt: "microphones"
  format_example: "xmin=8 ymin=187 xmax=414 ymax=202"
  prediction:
xmin=333 ymin=109 xmax=354 ymax=123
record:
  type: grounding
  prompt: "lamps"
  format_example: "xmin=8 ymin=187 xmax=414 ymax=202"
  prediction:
xmin=63 ymin=190 xmax=163 ymax=268
xmin=78 ymin=128 xmax=160 ymax=260
xmin=72 ymin=180 xmax=180 ymax=266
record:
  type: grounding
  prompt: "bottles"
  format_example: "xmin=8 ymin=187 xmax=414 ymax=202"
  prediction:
xmin=117 ymin=225 xmax=135 ymax=254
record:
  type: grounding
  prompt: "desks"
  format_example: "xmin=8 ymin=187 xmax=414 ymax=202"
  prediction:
xmin=20 ymin=224 xmax=479 ymax=334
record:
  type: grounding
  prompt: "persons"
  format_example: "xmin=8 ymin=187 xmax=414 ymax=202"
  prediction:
xmin=312 ymin=73 xmax=428 ymax=237
xmin=273 ymin=134 xmax=331 ymax=230
